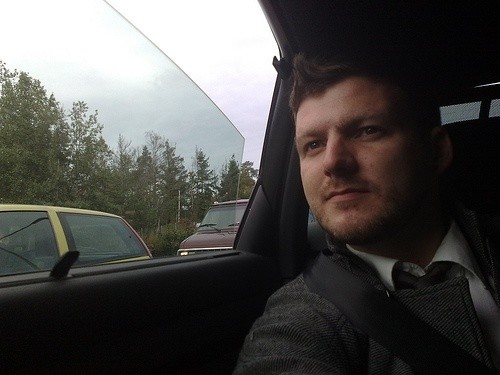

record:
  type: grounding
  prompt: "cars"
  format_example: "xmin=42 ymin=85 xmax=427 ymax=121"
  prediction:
xmin=0 ymin=204 xmax=154 ymax=276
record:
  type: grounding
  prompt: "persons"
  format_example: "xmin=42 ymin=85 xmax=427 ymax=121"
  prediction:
xmin=235 ymin=45 xmax=500 ymax=375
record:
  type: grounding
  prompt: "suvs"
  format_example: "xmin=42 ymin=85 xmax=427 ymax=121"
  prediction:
xmin=177 ymin=198 xmax=249 ymax=257
